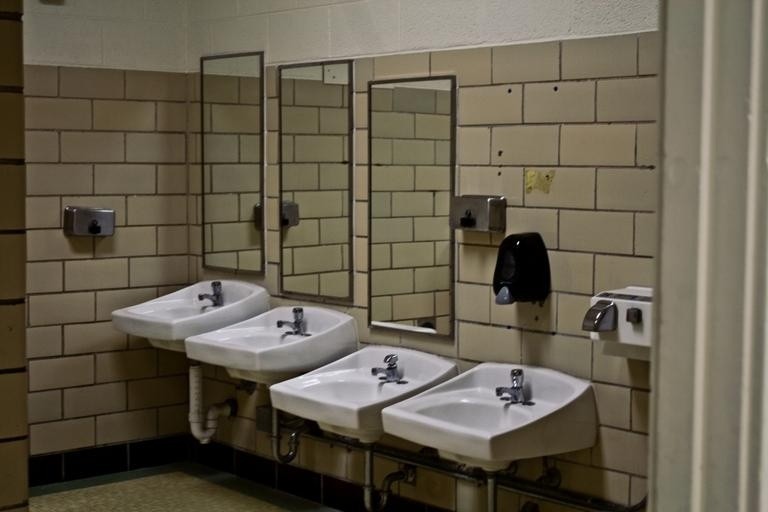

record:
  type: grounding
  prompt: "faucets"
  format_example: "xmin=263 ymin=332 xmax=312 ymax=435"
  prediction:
xmin=277 ymin=307 xmax=305 ymax=335
xmin=495 ymin=368 xmax=527 ymax=403
xmin=198 ymin=281 xmax=224 ymax=306
xmin=370 ymin=352 xmax=402 ymax=381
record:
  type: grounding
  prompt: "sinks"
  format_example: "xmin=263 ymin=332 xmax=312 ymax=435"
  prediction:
xmin=183 ymin=305 xmax=359 ymax=386
xmin=269 ymin=343 xmax=461 ymax=431
xmin=381 ymin=361 xmax=601 ymax=460
xmin=110 ymin=280 xmax=271 ymax=351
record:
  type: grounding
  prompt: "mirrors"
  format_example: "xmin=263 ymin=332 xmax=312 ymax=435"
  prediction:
xmin=367 ymin=74 xmax=455 ymax=340
xmin=199 ymin=50 xmax=264 ymax=275
xmin=277 ymin=59 xmax=354 ymax=306
